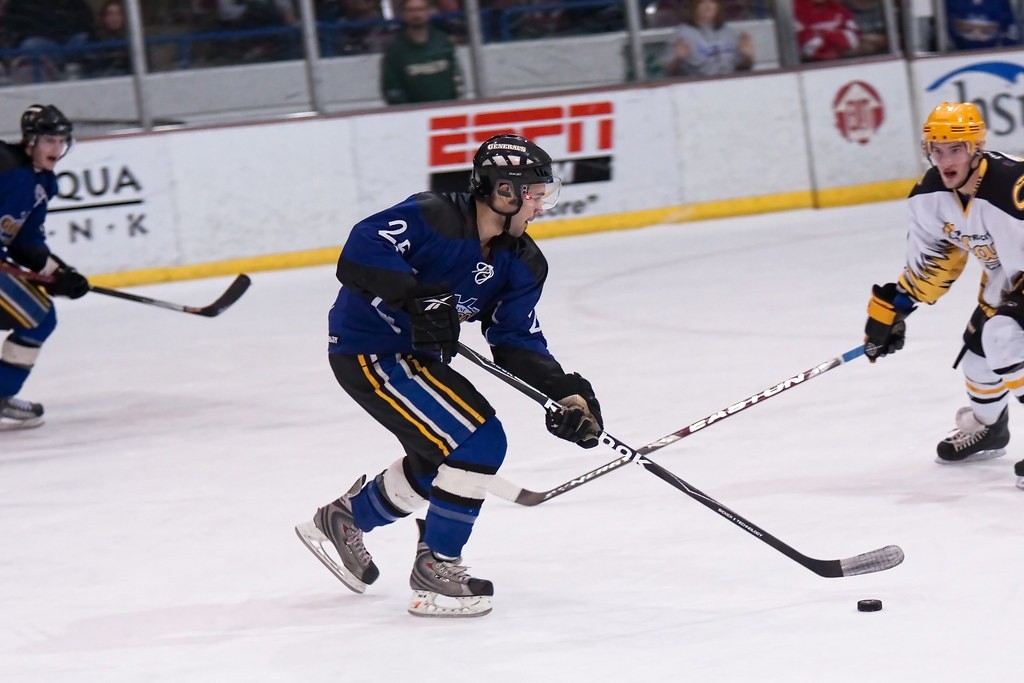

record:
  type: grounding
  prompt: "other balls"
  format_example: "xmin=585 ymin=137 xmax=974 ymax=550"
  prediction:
xmin=857 ymin=598 xmax=883 ymax=612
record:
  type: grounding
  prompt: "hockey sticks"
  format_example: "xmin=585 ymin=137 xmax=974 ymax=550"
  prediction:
xmin=456 ymin=340 xmax=905 ymax=579
xmin=1 ymin=261 xmax=252 ymax=319
xmin=485 ymin=344 xmax=868 ymax=509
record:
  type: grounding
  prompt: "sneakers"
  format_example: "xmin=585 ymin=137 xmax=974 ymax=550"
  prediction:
xmin=937 ymin=404 xmax=1010 ymax=460
xmin=1015 ymin=459 xmax=1024 ymax=489
xmin=295 ymin=474 xmax=379 ymax=594
xmin=408 ymin=519 xmax=494 ymax=615
xmin=0 ymin=397 xmax=47 ymax=428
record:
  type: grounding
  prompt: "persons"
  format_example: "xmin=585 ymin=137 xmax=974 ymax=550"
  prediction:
xmin=378 ymin=1 xmax=480 ymax=108
xmin=783 ymin=1 xmax=864 ymax=63
xmin=294 ymin=135 xmax=602 ymax=618
xmin=860 ymin=99 xmax=1022 ymax=496
xmin=0 ymin=103 xmax=90 ymax=421
xmin=7 ymin=2 xmax=622 ymax=76
xmin=928 ymin=1 xmax=1020 ymax=55
xmin=651 ymin=1 xmax=757 ymax=82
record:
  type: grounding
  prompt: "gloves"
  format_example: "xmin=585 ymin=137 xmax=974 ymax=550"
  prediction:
xmin=34 ymin=255 xmax=89 ymax=299
xmin=406 ymin=292 xmax=462 ymax=369
xmin=545 ymin=371 xmax=605 ymax=449
xmin=864 ymin=281 xmax=922 ymax=363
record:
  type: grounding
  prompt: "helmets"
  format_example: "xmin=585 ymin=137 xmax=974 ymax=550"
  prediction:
xmin=921 ymin=101 xmax=987 ymax=156
xmin=472 ymin=134 xmax=554 ymax=184
xmin=21 ymin=103 xmax=73 ymax=135
